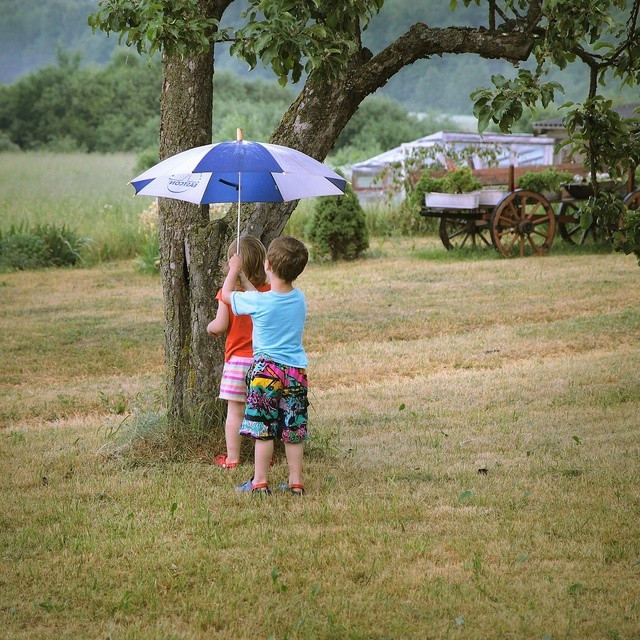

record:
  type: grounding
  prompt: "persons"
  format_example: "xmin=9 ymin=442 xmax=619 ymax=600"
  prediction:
xmin=206 ymin=236 xmax=276 ymax=468
xmin=221 ymin=236 xmax=309 ymax=495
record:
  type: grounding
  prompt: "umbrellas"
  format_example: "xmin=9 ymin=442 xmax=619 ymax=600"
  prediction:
xmin=127 ymin=127 xmax=351 ymax=253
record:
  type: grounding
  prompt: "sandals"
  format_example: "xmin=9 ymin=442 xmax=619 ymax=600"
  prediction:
xmin=279 ymin=483 xmax=305 ymax=496
xmin=235 ymin=477 xmax=272 ymax=495
xmin=215 ymin=455 xmax=239 ymax=468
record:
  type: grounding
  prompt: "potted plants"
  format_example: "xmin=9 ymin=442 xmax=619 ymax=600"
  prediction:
xmin=414 ymin=167 xmax=483 ymax=209
xmin=519 ymin=171 xmax=562 ymax=202
